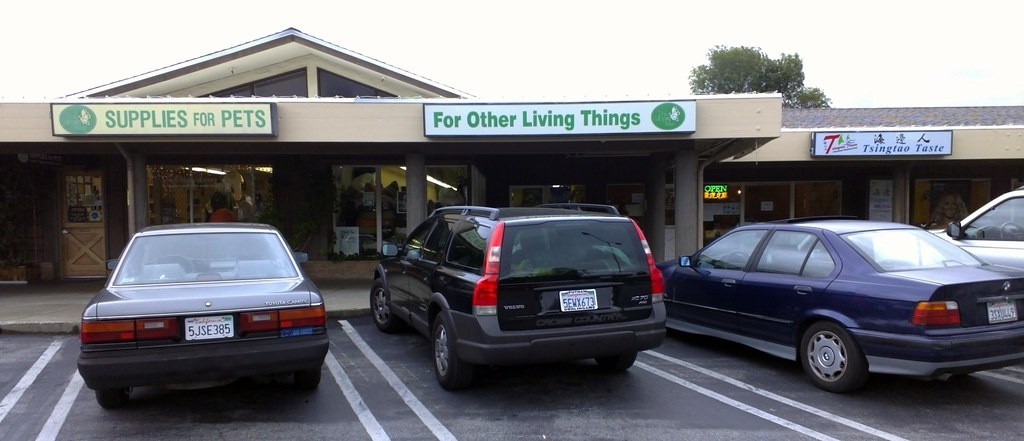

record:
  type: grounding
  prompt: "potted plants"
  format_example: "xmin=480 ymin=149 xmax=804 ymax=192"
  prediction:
xmin=0 ymin=192 xmax=33 ymax=283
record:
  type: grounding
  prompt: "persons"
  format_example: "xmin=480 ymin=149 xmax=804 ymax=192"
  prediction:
xmin=210 ymin=191 xmax=235 ymax=222
xmin=615 ymin=198 xmax=628 ymax=214
xmin=930 ymin=191 xmax=968 ymax=223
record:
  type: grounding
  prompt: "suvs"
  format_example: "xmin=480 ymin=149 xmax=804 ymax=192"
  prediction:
xmin=881 ymin=186 xmax=1024 ymax=267
xmin=371 ymin=203 xmax=669 ymax=392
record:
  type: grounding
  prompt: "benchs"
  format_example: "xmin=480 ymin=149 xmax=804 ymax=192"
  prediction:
xmin=137 ymin=260 xmax=288 ymax=280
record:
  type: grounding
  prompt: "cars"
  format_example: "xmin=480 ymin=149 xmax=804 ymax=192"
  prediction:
xmin=656 ymin=220 xmax=1024 ymax=395
xmin=78 ymin=223 xmax=329 ymax=413
